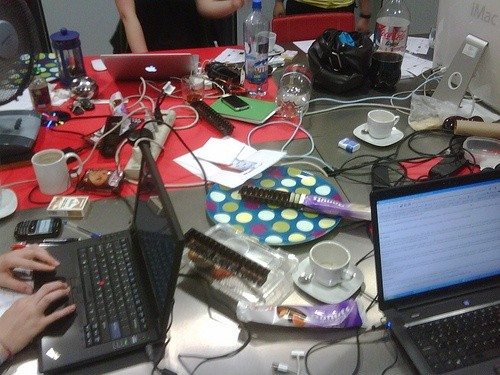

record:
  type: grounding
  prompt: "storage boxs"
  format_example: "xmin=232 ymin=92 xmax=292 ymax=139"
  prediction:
xmin=47 ymin=195 xmax=90 ymax=219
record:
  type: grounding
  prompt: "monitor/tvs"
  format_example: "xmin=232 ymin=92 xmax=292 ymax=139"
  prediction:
xmin=430 ymin=0 xmax=500 ymax=124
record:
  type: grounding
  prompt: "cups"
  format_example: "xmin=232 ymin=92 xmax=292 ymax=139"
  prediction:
xmin=367 ymin=110 xmax=400 ymax=139
xmin=31 ymin=148 xmax=82 ymax=194
xmin=309 ymin=239 xmax=356 ymax=287
xmin=180 ymin=75 xmax=205 ymax=106
xmin=258 ymin=31 xmax=277 ymax=52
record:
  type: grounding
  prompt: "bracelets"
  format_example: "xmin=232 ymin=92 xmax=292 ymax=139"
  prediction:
xmin=0 ymin=339 xmax=13 ymax=363
xmin=359 ymin=13 xmax=372 ymax=20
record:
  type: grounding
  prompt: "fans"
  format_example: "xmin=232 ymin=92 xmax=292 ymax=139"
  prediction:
xmin=0 ymin=0 xmax=43 ymax=165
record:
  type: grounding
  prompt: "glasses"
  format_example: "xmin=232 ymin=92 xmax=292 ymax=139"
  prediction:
xmin=441 ymin=115 xmax=484 ymax=130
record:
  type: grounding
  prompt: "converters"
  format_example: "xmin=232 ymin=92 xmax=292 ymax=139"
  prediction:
xmin=371 ymin=166 xmax=389 ymax=190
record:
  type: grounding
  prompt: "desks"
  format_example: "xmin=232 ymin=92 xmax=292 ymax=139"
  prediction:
xmin=0 ymin=33 xmax=500 ymax=375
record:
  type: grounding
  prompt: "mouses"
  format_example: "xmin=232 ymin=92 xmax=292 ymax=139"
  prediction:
xmin=91 ymin=59 xmax=107 ymax=71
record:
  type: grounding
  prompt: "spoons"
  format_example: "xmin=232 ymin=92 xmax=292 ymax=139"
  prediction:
xmin=298 ymin=272 xmax=312 ymax=284
xmin=360 ymin=129 xmax=368 ymax=134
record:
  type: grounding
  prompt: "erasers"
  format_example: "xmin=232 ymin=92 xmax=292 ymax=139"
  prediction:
xmin=338 ymin=137 xmax=360 ymax=152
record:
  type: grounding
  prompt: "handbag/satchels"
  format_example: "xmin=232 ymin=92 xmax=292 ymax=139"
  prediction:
xmin=308 ymin=28 xmax=372 ymax=97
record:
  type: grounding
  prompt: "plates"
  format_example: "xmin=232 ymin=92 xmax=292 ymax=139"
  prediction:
xmin=0 ymin=188 xmax=17 ymax=218
xmin=292 ymin=256 xmax=363 ymax=305
xmin=206 ymin=166 xmax=343 ymax=246
xmin=269 ymin=44 xmax=284 ymax=57
xmin=352 ymin=123 xmax=405 ymax=146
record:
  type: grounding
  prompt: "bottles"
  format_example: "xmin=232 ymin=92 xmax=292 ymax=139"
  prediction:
xmin=368 ymin=0 xmax=411 ymax=88
xmin=28 ymin=68 xmax=52 ymax=113
xmin=273 ymin=63 xmax=313 ymax=118
xmin=243 ymin=0 xmax=270 ymax=96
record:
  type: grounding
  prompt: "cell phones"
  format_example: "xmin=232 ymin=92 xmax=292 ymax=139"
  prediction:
xmin=15 ymin=218 xmax=63 ymax=241
xmin=221 ymin=95 xmax=249 ymax=110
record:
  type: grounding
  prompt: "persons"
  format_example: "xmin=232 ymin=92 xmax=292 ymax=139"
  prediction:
xmin=109 ymin=0 xmax=247 ymax=54
xmin=273 ymin=0 xmax=372 ymax=37
xmin=0 ymin=243 xmax=76 ymax=364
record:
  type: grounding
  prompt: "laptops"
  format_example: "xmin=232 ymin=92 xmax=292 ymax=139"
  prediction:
xmin=36 ymin=142 xmax=185 ymax=375
xmin=99 ymin=53 xmax=198 ymax=82
xmin=368 ymin=168 xmax=500 ymax=375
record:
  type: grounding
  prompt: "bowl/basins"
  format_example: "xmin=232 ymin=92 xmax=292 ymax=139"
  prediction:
xmin=463 ymin=137 xmax=500 ymax=168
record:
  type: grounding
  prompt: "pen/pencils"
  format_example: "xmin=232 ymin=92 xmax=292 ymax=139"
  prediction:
xmin=64 ymin=221 xmax=99 ymax=237
xmin=10 ymin=244 xmax=51 ymax=249
xmin=43 ymin=238 xmax=78 ymax=242
